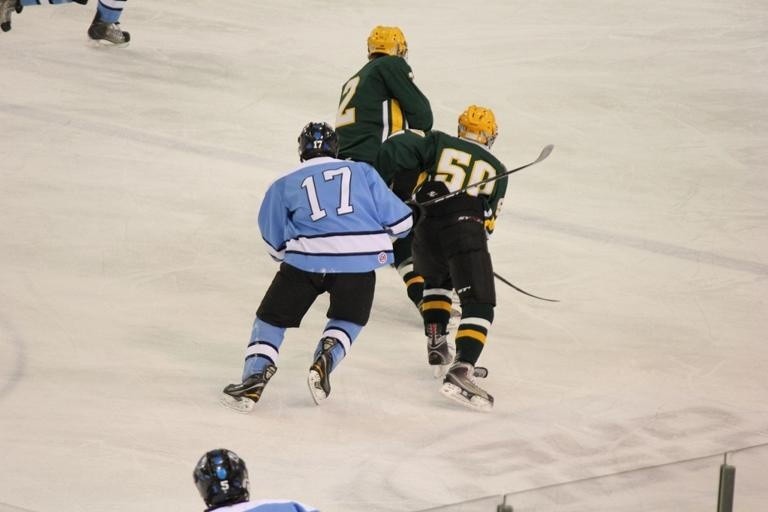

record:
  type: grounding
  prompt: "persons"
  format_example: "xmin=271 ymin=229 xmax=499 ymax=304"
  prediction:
xmin=376 ymin=102 xmax=510 ymax=405
xmin=334 ymin=23 xmax=464 ymax=319
xmin=218 ymin=119 xmax=420 ymax=404
xmin=191 ymin=449 xmax=325 ymax=512
xmin=0 ymin=0 xmax=130 ymax=49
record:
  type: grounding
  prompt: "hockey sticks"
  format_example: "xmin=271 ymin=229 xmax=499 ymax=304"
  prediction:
xmin=420 ymin=145 xmax=553 ymax=207
xmin=493 ymin=273 xmax=561 ymax=303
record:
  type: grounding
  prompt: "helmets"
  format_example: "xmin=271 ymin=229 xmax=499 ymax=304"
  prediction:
xmin=367 ymin=25 xmax=408 ymax=59
xmin=192 ymin=448 xmax=250 ymax=508
xmin=457 ymin=104 xmax=498 ymax=148
xmin=297 ymin=122 xmax=339 ymax=162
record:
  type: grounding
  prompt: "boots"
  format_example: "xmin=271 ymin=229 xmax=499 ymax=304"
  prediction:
xmin=441 ymin=362 xmax=496 ymax=403
xmin=222 ymin=373 xmax=269 ymax=404
xmin=309 ymin=351 xmax=331 ymax=397
xmin=427 ymin=335 xmax=456 ymax=364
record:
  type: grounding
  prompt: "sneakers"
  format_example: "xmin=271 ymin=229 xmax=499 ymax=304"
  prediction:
xmin=88 ymin=19 xmax=131 ymax=44
xmin=0 ymin=1 xmax=18 ymax=31
